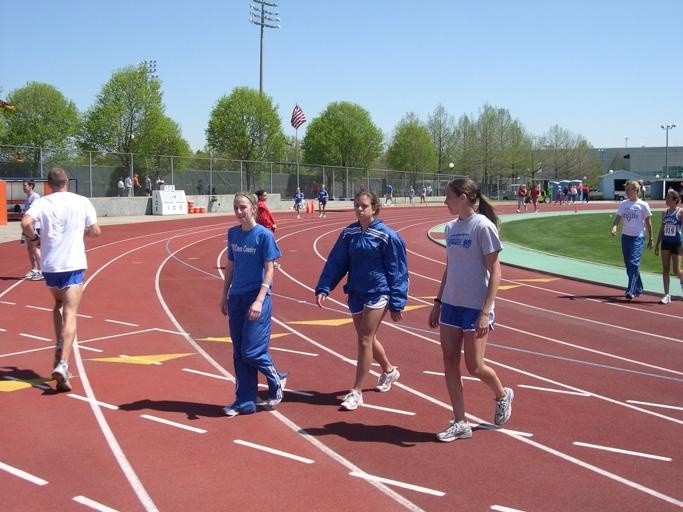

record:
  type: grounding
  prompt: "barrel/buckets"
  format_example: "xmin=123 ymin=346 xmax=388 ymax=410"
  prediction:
xmin=194 ymin=208 xmax=200 ymax=213
xmin=200 ymin=207 xmax=205 ymax=213
xmin=188 ymin=201 xmax=194 ymax=213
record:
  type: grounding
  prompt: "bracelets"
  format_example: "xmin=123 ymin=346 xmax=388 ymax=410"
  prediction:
xmin=261 ymin=283 xmax=269 ymax=289
xmin=434 ymin=298 xmax=441 ymax=303
xmin=649 ymin=237 xmax=653 ymax=241
xmin=30 ymin=234 xmax=39 ymax=241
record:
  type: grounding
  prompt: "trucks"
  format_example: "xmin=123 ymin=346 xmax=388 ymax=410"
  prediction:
xmin=613 ymin=184 xmax=652 ymax=201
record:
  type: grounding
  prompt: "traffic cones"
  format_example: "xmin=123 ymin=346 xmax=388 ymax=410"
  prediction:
xmin=303 ymin=202 xmax=310 ymax=214
xmin=310 ymin=199 xmax=317 ymax=214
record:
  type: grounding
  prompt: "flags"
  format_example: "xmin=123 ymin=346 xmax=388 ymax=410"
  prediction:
xmin=291 ymin=105 xmax=307 ymax=129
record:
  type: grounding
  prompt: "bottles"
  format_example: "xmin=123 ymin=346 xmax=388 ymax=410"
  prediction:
xmin=19 ymin=233 xmax=25 ymax=246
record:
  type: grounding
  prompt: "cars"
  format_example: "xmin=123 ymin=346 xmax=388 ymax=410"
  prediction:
xmin=501 ymin=183 xmax=522 ymax=201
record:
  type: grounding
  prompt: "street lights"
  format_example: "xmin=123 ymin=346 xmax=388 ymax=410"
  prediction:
xmin=448 ymin=162 xmax=454 ymax=175
xmin=659 ymin=123 xmax=675 ymax=178
xmin=598 ymin=147 xmax=607 ymax=174
xmin=623 ymin=135 xmax=630 ymax=148
xmin=247 ymin=0 xmax=282 ymax=166
xmin=133 ymin=58 xmax=159 ymax=127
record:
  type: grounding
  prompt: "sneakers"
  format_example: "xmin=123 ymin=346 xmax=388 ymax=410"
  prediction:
xmin=268 ymin=377 xmax=287 ymax=406
xmin=24 ymin=269 xmax=45 ymax=281
xmin=340 ymin=389 xmax=363 ymax=410
xmin=51 ymin=364 xmax=71 ymax=392
xmin=625 ymin=292 xmax=641 ymax=299
xmin=494 ymin=387 xmax=514 ymax=425
xmin=55 ymin=342 xmax=62 ymax=359
xmin=659 ymin=294 xmax=671 ymax=304
xmin=375 ymin=366 xmax=400 ymax=392
xmin=223 ymin=406 xmax=256 ymax=416
xmin=435 ymin=418 xmax=472 ymax=442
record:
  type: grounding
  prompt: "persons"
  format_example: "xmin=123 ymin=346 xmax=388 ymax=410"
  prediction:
xmin=21 ymin=179 xmax=44 ymax=280
xmin=609 ymin=181 xmax=654 ymax=299
xmin=515 ymin=182 xmax=589 ymax=214
xmin=384 ymin=184 xmax=393 ymax=205
xmin=118 ymin=173 xmax=164 ymax=197
xmin=219 ymin=192 xmax=287 ymax=416
xmin=293 ymin=188 xmax=304 ymax=219
xmin=408 ymin=183 xmax=433 ymax=206
xmin=655 ymin=191 xmax=683 ymax=304
xmin=311 ymin=180 xmax=319 ymax=198
xmin=22 ymin=168 xmax=98 ymax=391
xmin=256 ymin=190 xmax=281 ymax=269
xmin=314 ymin=182 xmax=409 ymax=410
xmin=429 ymin=179 xmax=514 ymax=443
xmin=317 ymin=185 xmax=328 ymax=218
